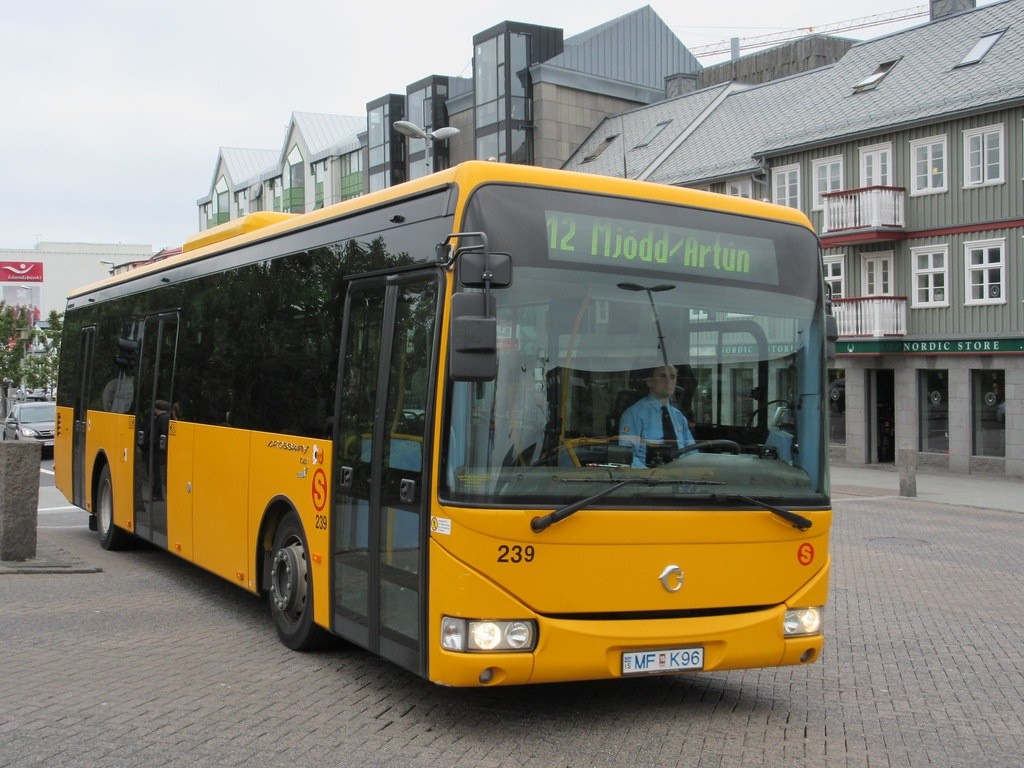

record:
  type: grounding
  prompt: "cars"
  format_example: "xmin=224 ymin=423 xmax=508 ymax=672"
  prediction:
xmin=12 ymin=385 xmax=57 ymax=401
xmin=829 ymin=378 xmax=893 ymax=423
xmin=2 ymin=402 xmax=57 ymax=447
xmin=928 ymin=389 xmax=948 ymax=419
xmin=976 ymin=394 xmax=1006 ymax=424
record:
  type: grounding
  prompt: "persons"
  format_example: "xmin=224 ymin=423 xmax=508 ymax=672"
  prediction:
xmin=150 ymin=400 xmax=185 ymax=500
xmin=620 ymin=361 xmax=696 ymax=469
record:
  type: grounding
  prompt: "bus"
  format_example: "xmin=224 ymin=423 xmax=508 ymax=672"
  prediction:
xmin=50 ymin=158 xmax=834 ymax=702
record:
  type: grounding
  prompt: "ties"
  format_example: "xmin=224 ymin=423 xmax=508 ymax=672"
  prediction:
xmin=661 ymin=405 xmax=679 ymax=459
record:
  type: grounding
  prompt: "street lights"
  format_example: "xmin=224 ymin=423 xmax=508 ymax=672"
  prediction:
xmin=391 ymin=120 xmax=462 ymax=175
xmin=21 ymin=285 xmax=34 ymax=328
xmin=100 ymin=260 xmax=116 ymax=277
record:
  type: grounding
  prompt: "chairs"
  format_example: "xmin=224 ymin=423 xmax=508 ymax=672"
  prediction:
xmin=608 ymin=391 xmax=645 ymax=437
